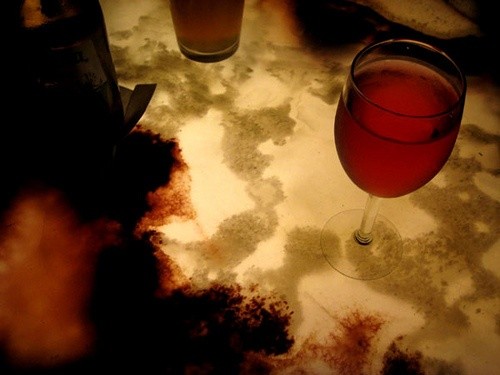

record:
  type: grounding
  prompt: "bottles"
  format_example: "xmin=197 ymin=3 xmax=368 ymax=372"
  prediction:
xmin=1 ymin=0 xmax=125 ymax=141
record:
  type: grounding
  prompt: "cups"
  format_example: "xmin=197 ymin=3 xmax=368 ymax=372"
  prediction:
xmin=169 ymin=0 xmax=245 ymax=63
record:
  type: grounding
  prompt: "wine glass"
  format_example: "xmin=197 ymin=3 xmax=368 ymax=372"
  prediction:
xmin=318 ymin=35 xmax=468 ymax=281
